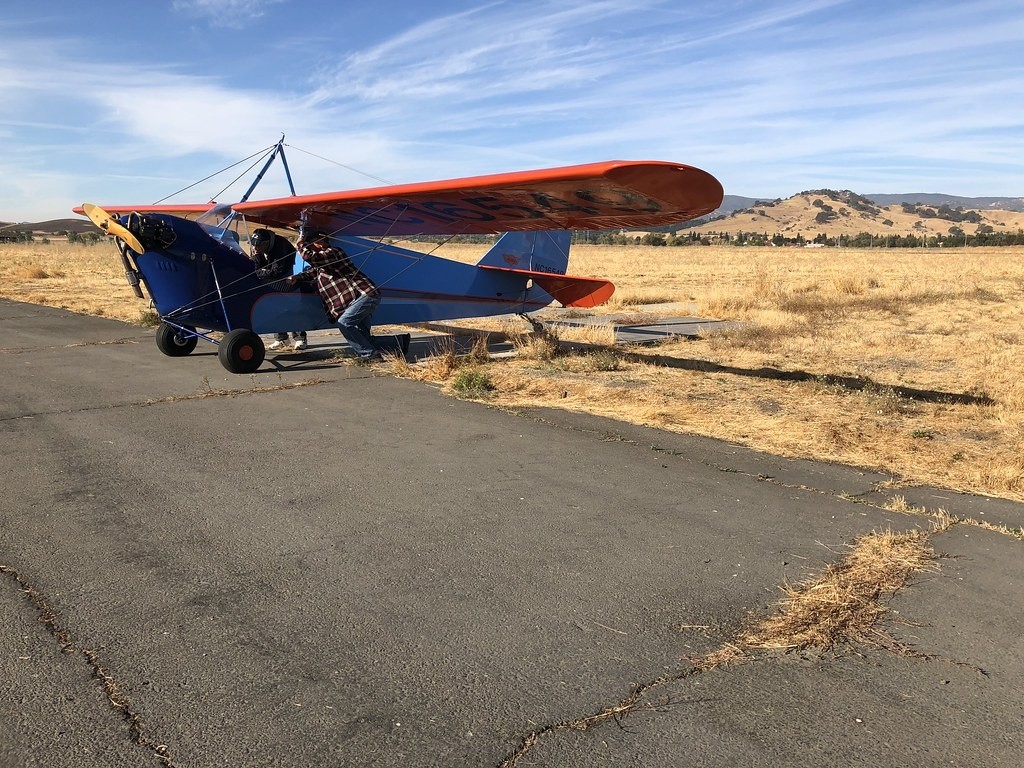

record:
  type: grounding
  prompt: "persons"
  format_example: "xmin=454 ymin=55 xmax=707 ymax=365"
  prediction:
xmin=248 ymin=228 xmax=309 ymax=350
xmin=296 ymin=218 xmax=412 ymax=367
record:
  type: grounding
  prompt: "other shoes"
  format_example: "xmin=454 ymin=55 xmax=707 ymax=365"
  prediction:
xmin=356 ymin=353 xmax=382 ymax=364
xmin=397 ymin=333 xmax=410 ymax=358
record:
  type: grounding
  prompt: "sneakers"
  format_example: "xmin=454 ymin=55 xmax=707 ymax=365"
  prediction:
xmin=294 ymin=339 xmax=307 ymax=349
xmin=269 ymin=338 xmax=291 ymax=349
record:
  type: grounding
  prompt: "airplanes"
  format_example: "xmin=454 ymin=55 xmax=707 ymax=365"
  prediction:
xmin=71 ymin=130 xmax=725 ymax=376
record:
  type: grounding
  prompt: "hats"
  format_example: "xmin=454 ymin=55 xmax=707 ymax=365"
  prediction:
xmin=247 ymin=229 xmax=270 ymax=245
